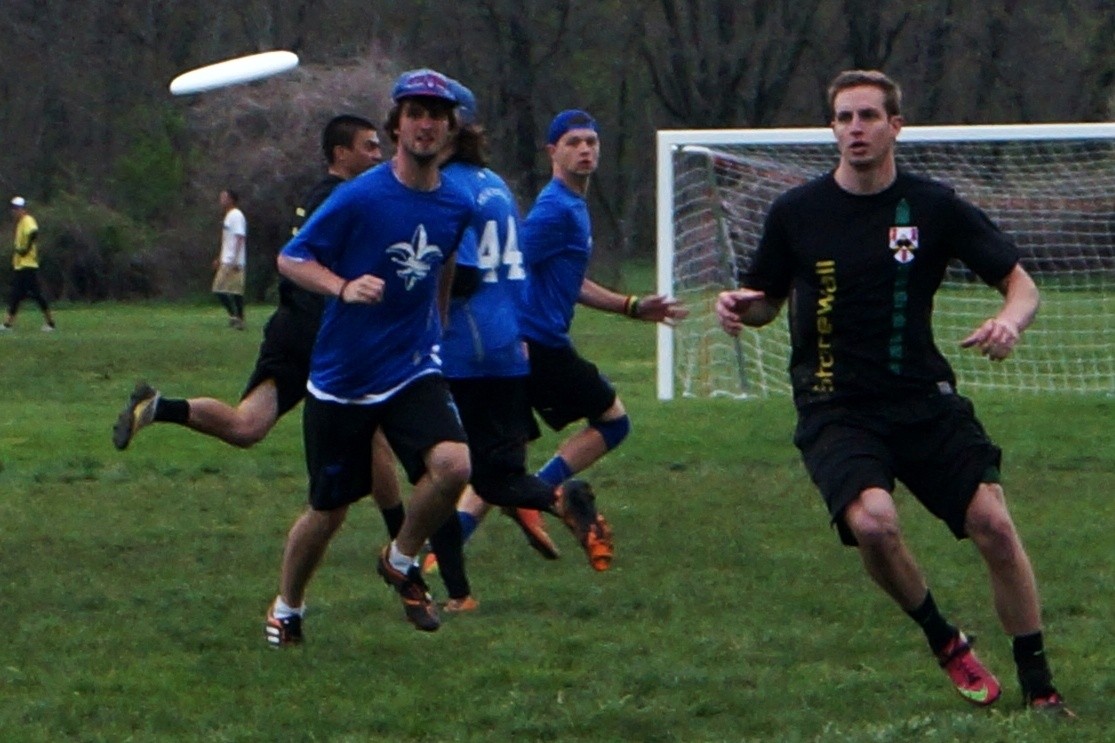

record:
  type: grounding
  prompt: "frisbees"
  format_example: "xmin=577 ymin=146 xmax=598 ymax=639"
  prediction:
xmin=168 ymin=49 xmax=300 ymax=95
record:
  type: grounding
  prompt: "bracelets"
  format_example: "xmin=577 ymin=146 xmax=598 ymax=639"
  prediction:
xmin=623 ymin=294 xmax=638 ymax=317
xmin=340 ymin=280 xmax=349 ymax=299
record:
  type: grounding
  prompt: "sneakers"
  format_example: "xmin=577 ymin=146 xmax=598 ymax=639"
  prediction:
xmin=377 ymin=544 xmax=440 ymax=632
xmin=264 ymin=604 xmax=305 ymax=651
xmin=933 ymin=629 xmax=1001 ymax=706
xmin=112 ymin=380 xmax=160 ymax=451
xmin=1032 ymin=695 xmax=1076 ymax=719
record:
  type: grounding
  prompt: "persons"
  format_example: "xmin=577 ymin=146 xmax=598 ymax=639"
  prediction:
xmin=211 ymin=188 xmax=247 ymax=330
xmin=114 ymin=79 xmax=690 ymax=611
xmin=266 ymin=67 xmax=477 ymax=645
xmin=0 ymin=196 xmax=56 ymax=333
xmin=715 ymin=70 xmax=1080 ymax=721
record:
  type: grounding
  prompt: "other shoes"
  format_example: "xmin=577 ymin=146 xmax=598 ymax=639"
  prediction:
xmin=552 ymin=479 xmax=614 ymax=571
xmin=498 ymin=503 xmax=561 ymax=562
xmin=41 ymin=325 xmax=55 ymax=332
xmin=423 ymin=551 xmax=439 ymax=577
xmin=227 ymin=317 xmax=236 ymax=330
xmin=0 ymin=324 xmax=14 ymax=332
xmin=443 ymin=596 xmax=477 ymax=613
xmin=236 ymin=320 xmax=244 ymax=329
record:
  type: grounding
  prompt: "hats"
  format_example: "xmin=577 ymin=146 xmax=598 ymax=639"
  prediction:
xmin=547 ymin=110 xmax=599 ymax=146
xmin=392 ymin=68 xmax=459 ymax=108
xmin=448 ymin=77 xmax=476 ymax=123
xmin=9 ymin=196 xmax=25 ymax=207
